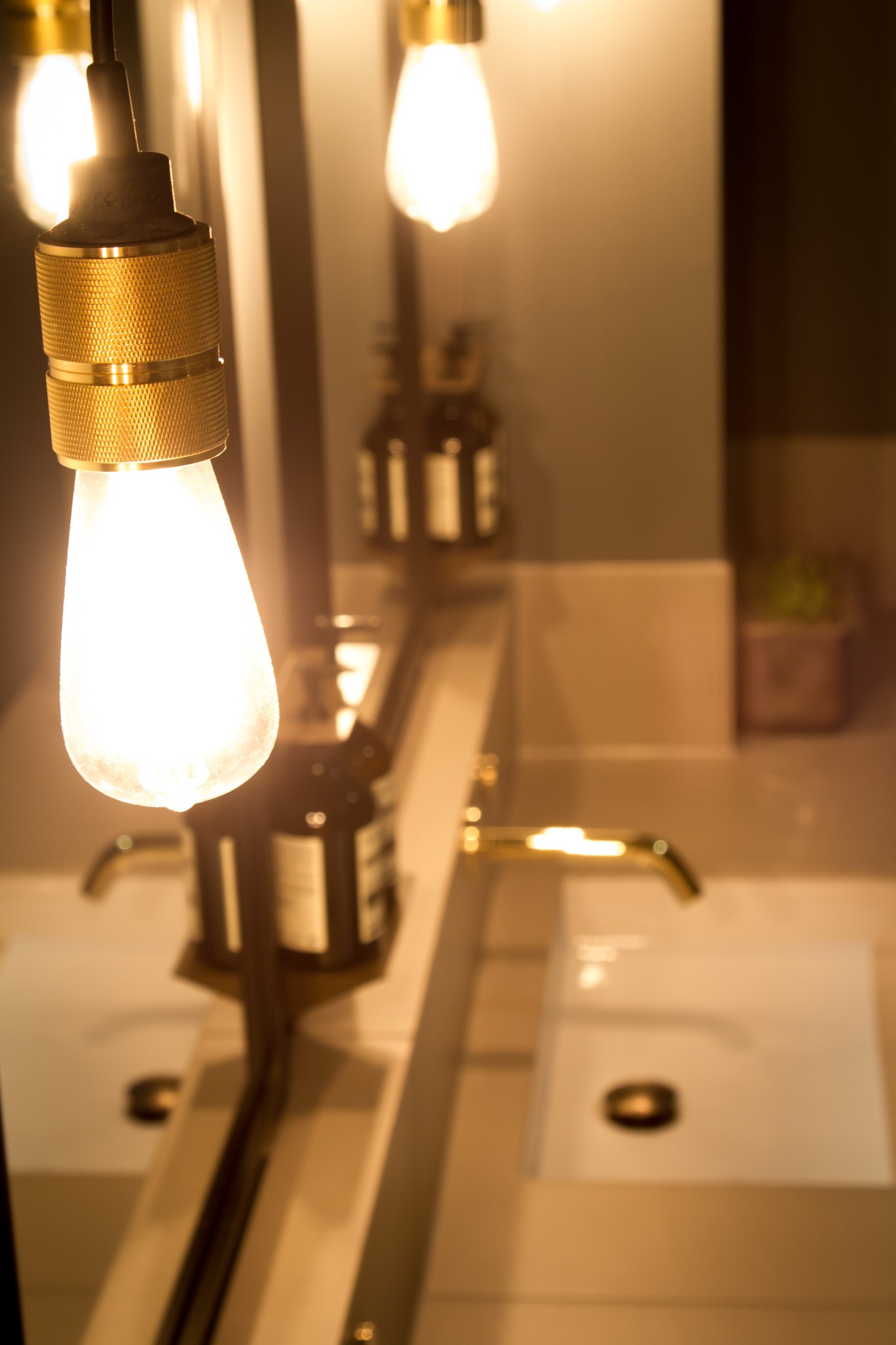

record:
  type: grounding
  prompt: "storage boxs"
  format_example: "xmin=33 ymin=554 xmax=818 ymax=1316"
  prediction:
xmin=742 ymin=561 xmax=861 ymax=732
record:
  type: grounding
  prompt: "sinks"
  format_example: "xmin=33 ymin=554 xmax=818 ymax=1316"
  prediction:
xmin=526 ymin=872 xmax=893 ymax=1191
xmin=2 ymin=863 xmax=224 ymax=1184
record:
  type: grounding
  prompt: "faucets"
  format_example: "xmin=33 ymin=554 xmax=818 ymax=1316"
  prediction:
xmin=82 ymin=832 xmax=180 ymax=902
xmin=465 ymin=805 xmax=704 ymax=908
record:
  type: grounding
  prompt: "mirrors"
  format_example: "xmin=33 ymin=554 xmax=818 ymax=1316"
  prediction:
xmin=1 ymin=0 xmax=435 ymax=1345
